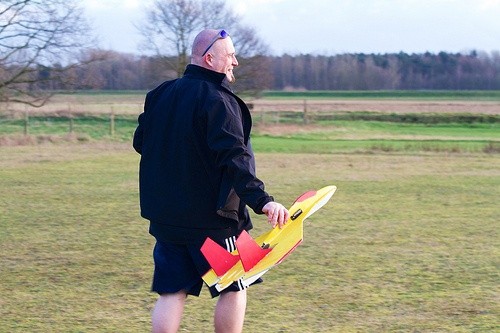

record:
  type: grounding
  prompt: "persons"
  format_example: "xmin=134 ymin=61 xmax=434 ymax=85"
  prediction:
xmin=132 ymin=30 xmax=289 ymax=333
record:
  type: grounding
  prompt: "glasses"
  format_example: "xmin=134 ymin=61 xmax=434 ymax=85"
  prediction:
xmin=201 ymin=30 xmax=229 ymax=57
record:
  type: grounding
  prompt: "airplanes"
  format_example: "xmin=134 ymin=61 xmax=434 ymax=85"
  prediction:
xmin=201 ymin=185 xmax=339 ymax=299
